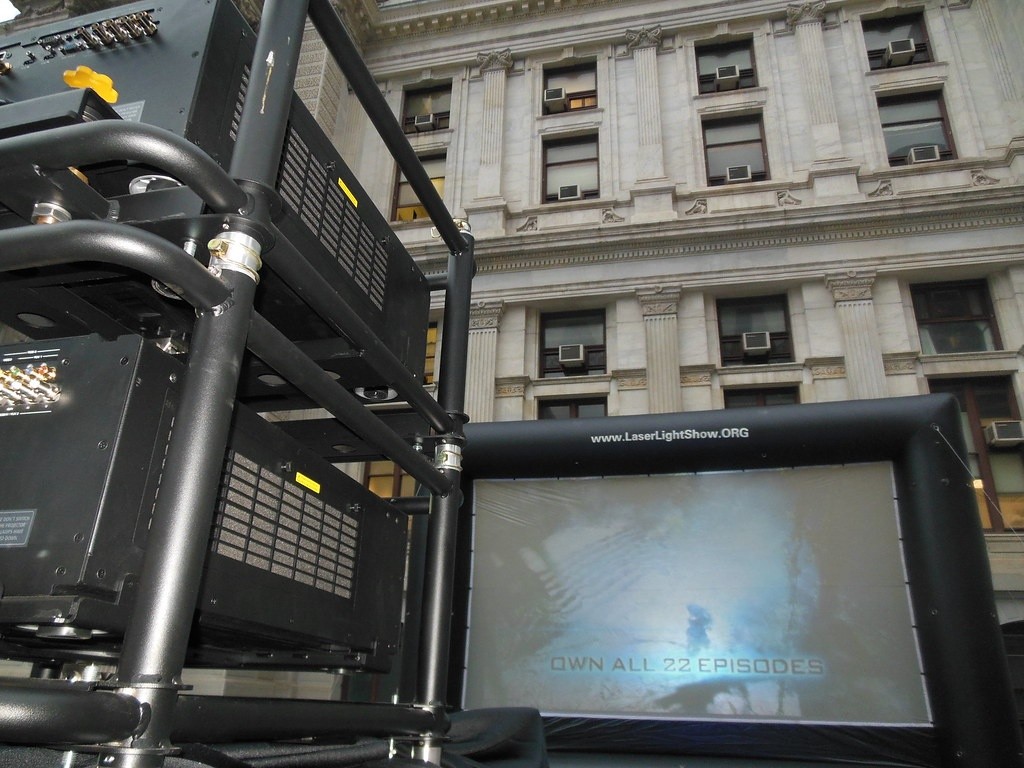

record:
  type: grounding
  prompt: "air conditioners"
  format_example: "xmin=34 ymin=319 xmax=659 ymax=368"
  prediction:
xmin=558 ymin=184 xmax=584 ymax=201
xmin=544 ymin=87 xmax=569 ymax=112
xmin=985 ymin=420 xmax=1024 ymax=447
xmin=716 ymin=64 xmax=740 ymax=90
xmin=741 ymin=331 xmax=771 ymax=355
xmin=559 ymin=343 xmax=585 ymax=363
xmin=907 ymin=145 xmax=940 ymax=165
xmin=886 ymin=38 xmax=916 ymax=66
xmin=726 ymin=164 xmax=752 ymax=183
xmin=415 ymin=113 xmax=436 ymax=131
